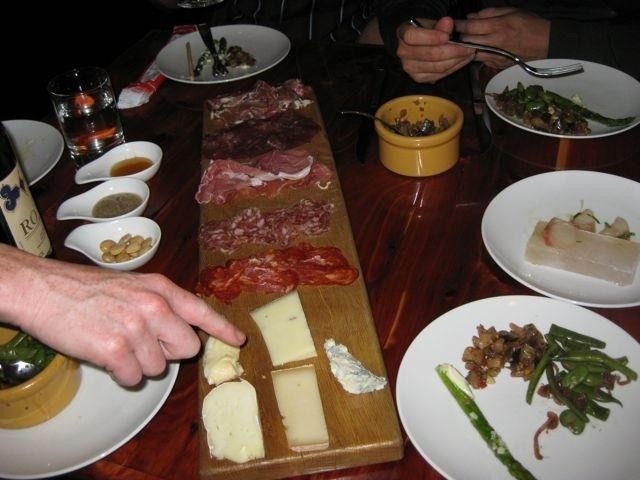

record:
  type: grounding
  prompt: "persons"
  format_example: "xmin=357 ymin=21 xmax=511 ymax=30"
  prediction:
xmin=0 ymin=242 xmax=248 ymax=388
xmin=155 ymin=0 xmax=384 ymax=46
xmin=376 ymin=1 xmax=639 ymax=103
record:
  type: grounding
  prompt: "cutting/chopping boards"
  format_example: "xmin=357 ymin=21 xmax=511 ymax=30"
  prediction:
xmin=195 ymin=79 xmax=403 ymax=480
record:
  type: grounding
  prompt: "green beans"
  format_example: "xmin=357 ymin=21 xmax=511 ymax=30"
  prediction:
xmin=0 ymin=333 xmax=55 ymax=392
xmin=526 ymin=324 xmax=636 ymax=434
xmin=437 ymin=364 xmax=538 ymax=480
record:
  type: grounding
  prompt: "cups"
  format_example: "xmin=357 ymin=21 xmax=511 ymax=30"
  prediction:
xmin=48 ymin=68 xmax=127 ymax=168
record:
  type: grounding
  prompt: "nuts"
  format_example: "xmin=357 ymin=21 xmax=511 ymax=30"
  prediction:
xmin=100 ymin=235 xmax=151 ymax=263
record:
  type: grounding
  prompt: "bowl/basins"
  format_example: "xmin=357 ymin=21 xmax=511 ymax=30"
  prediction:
xmin=374 ymin=94 xmax=464 ymax=178
xmin=0 ymin=324 xmax=85 ymax=431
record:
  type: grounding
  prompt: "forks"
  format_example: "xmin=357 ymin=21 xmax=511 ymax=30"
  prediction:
xmin=410 ymin=18 xmax=584 ymax=77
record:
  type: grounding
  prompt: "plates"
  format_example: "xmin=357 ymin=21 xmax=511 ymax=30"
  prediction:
xmin=484 ymin=56 xmax=640 ymax=139
xmin=0 ymin=120 xmax=163 ymax=271
xmin=153 ymin=24 xmax=290 ymax=84
xmin=0 ymin=353 xmax=181 ymax=480
xmin=395 ymin=294 xmax=640 ymax=480
xmin=481 ymin=170 xmax=640 ymax=308
